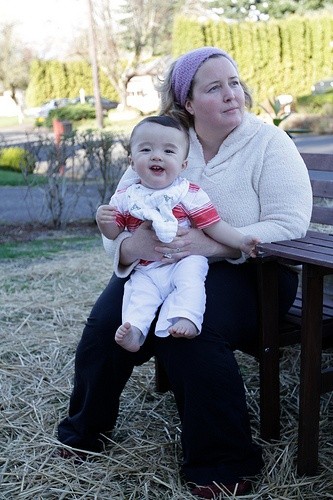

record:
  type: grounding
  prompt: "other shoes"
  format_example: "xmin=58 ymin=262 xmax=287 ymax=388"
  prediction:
xmin=185 ymin=479 xmax=254 ymax=499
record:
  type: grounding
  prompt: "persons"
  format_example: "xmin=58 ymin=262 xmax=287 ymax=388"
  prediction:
xmin=94 ymin=115 xmax=263 ymax=353
xmin=55 ymin=48 xmax=313 ymax=499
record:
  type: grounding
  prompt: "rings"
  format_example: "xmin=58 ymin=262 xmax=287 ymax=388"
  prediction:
xmin=176 ymin=248 xmax=180 ymax=253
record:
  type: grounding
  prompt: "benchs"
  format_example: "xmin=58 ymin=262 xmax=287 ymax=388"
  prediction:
xmin=154 ymin=153 xmax=333 ymax=442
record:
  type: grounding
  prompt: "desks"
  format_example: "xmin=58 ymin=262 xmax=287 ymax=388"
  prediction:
xmin=257 ymin=234 xmax=333 ymax=479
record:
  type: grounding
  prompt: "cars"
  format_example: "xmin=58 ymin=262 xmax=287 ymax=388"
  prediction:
xmin=72 ymin=96 xmax=118 ymax=110
xmin=37 ymin=98 xmax=74 ymax=119
